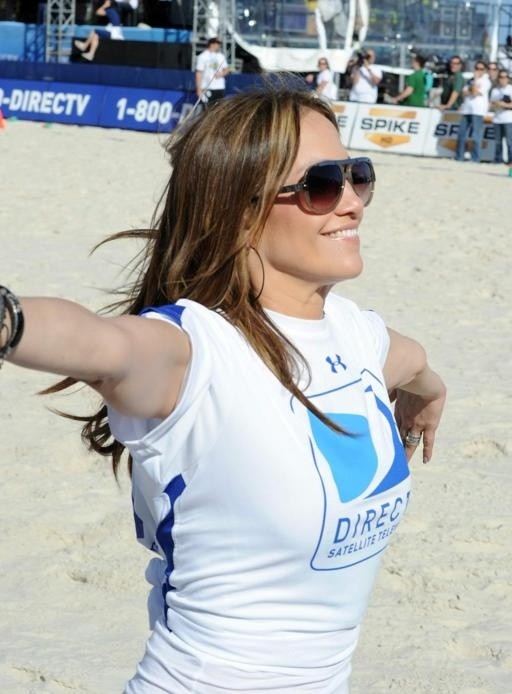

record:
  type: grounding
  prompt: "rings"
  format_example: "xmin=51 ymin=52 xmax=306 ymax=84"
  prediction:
xmin=406 ymin=431 xmax=421 ymax=444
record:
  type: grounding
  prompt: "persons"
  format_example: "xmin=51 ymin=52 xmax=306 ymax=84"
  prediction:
xmin=192 ymin=38 xmax=232 ymax=110
xmin=305 ymin=57 xmax=340 ymax=101
xmin=390 ymin=34 xmax=510 ymax=166
xmin=72 ymin=0 xmax=122 ymax=63
xmin=0 ymin=89 xmax=452 ymax=692
xmin=345 ymin=47 xmax=385 ymax=103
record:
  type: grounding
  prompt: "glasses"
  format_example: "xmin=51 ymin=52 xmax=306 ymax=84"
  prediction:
xmin=251 ymin=157 xmax=375 ymax=216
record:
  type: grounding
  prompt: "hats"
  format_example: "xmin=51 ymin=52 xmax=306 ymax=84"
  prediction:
xmin=208 ymin=37 xmax=224 ymax=45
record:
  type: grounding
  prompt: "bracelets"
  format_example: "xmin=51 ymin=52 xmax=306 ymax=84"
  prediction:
xmin=0 ymin=284 xmax=25 ymax=362
xmin=367 ymin=68 xmax=371 ymax=71
